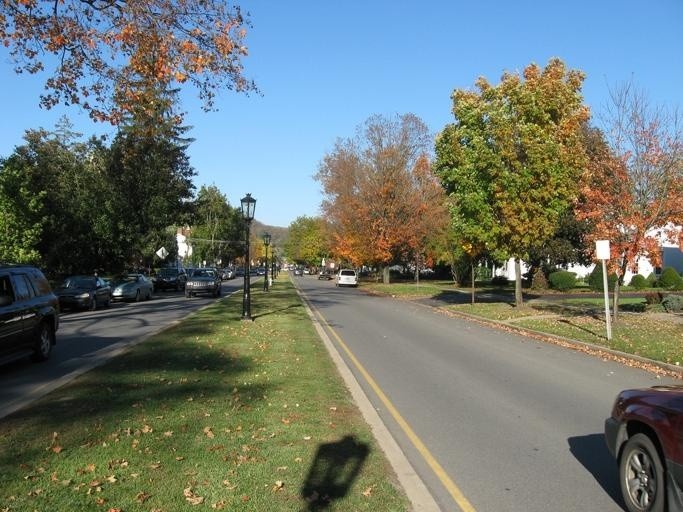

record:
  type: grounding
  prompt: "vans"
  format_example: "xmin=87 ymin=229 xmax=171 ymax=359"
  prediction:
xmin=1 ymin=264 xmax=59 ymax=371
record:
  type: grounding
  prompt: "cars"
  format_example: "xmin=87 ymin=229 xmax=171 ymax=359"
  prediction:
xmin=293 ymin=267 xmax=311 ymax=276
xmin=153 ymin=267 xmax=189 ymax=294
xmin=186 ymin=266 xmax=265 ymax=293
xmin=318 ymin=267 xmax=331 ymax=280
xmin=184 ymin=269 xmax=221 ymax=298
xmin=107 ymin=273 xmax=154 ymax=303
xmin=54 ymin=275 xmax=112 ymax=312
xmin=604 ymin=383 xmax=682 ymax=512
xmin=335 ymin=269 xmax=358 ymax=287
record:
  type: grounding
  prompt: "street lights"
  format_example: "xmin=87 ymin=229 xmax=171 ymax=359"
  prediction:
xmin=271 ymin=252 xmax=281 ymax=283
xmin=262 ymin=233 xmax=270 ymax=293
xmin=239 ymin=191 xmax=256 ymax=321
